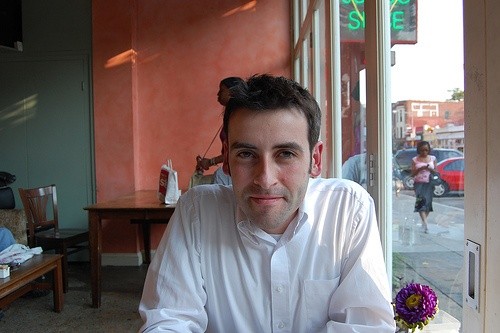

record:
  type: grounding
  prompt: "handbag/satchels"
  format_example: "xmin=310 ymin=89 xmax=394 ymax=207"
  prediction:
xmin=159 ymin=160 xmax=179 ymax=205
xmin=189 ymin=171 xmax=215 ymax=189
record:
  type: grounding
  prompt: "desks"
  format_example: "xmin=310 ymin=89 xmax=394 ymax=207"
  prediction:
xmin=0 ymin=254 xmax=64 ymax=313
xmin=83 ymin=190 xmax=185 ymax=308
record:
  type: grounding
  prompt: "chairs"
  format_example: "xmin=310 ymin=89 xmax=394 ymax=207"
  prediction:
xmin=18 ymin=183 xmax=90 ymax=293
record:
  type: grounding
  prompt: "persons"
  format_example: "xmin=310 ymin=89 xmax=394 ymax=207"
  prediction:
xmin=197 ymin=125 xmax=235 ymax=185
xmin=191 ymin=77 xmax=246 ymax=170
xmin=412 ymin=142 xmax=441 ymax=234
xmin=138 ymin=73 xmax=397 ymax=333
xmin=342 ymin=152 xmax=368 ymax=192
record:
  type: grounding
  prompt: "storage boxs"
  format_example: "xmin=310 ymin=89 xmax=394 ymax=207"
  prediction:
xmin=0 ymin=267 xmax=10 ymax=278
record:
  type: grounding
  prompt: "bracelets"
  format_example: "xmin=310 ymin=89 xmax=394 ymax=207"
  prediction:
xmin=211 ymin=157 xmax=216 ymax=166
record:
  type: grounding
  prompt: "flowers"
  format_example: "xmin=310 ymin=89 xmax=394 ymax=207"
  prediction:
xmin=390 ymin=278 xmax=440 ymax=333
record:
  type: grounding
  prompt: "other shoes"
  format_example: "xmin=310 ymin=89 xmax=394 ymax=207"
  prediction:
xmin=421 ymin=224 xmax=429 ymax=234
